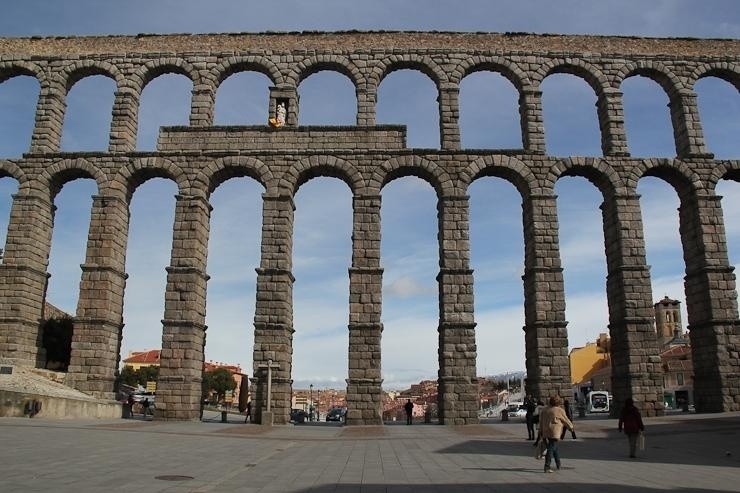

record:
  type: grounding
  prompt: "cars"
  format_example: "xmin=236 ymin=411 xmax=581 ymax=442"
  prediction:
xmin=326 ymin=408 xmax=347 ymax=422
xmin=128 ymin=391 xmax=155 ymax=406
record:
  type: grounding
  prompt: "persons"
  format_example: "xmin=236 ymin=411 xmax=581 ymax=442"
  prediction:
xmin=143 ymin=398 xmax=149 ymax=417
xmin=539 ymin=396 xmax=573 ymax=472
xmin=404 ymin=399 xmax=413 ymax=425
xmin=127 ymin=395 xmax=134 ymax=417
xmin=277 ymin=102 xmax=287 ymax=126
xmin=245 ymin=402 xmax=251 ymax=424
xmin=524 ymin=394 xmax=576 ymax=447
xmin=618 ymin=398 xmax=644 ymax=458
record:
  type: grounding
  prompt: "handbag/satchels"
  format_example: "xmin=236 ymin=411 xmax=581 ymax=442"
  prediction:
xmin=639 ymin=431 xmax=648 ymax=451
xmin=535 ymin=440 xmax=547 ymax=459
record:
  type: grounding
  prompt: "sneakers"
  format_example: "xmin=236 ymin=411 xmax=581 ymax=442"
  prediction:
xmin=545 ymin=469 xmax=553 ymax=473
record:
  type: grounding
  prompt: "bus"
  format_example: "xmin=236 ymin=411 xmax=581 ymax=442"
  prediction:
xmin=585 ymin=391 xmax=609 ymax=412
xmin=508 ymin=402 xmax=524 ymax=417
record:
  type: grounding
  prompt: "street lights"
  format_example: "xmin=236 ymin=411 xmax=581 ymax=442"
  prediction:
xmin=310 ymin=384 xmax=320 ymax=421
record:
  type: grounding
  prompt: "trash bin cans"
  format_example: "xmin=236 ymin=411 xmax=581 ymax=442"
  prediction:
xmin=28 ymin=399 xmax=42 ymax=418
xmin=222 ymin=411 xmax=227 ymax=422
xmin=425 ymin=412 xmax=431 ymax=423
xmin=501 ymin=409 xmax=508 ymax=421
xmin=578 ymin=407 xmax=585 ymax=417
xmin=681 ymin=400 xmax=689 ymax=412
